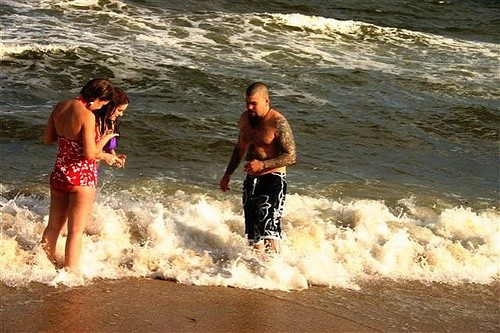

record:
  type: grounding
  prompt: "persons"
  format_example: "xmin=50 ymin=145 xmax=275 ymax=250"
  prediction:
xmin=41 ymin=78 xmax=129 ymax=274
xmin=219 ymin=83 xmax=297 ymax=253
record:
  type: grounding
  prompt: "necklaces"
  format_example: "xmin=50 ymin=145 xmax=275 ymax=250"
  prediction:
xmin=263 ymin=108 xmax=271 ymax=116
